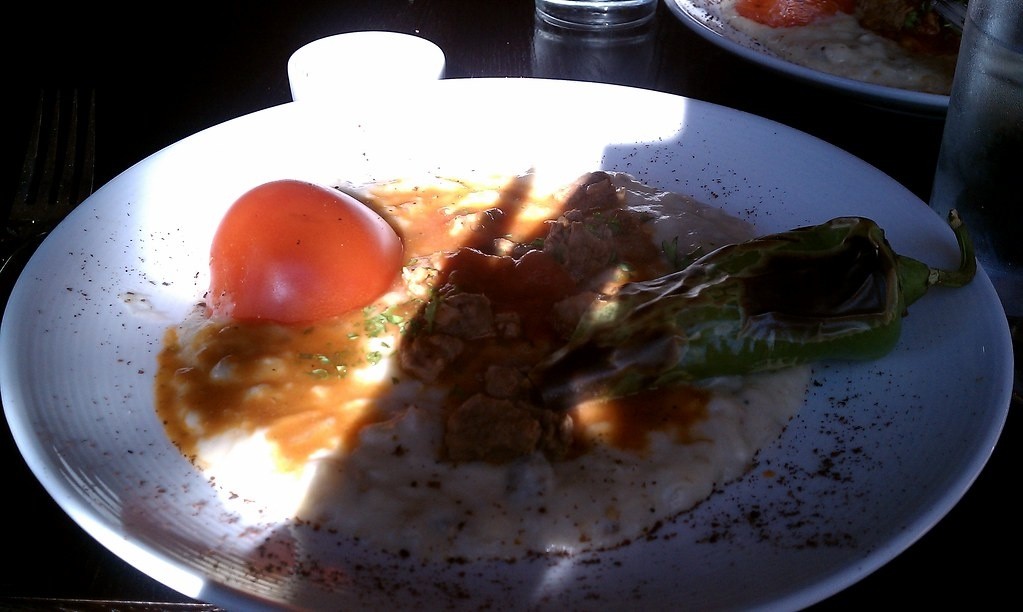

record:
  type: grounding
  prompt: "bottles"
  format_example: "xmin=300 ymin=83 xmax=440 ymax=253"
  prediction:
xmin=529 ymin=0 xmax=658 ymax=85
xmin=932 ymin=0 xmax=1023 ymax=325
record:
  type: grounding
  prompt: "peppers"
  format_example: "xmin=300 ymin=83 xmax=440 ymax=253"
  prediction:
xmin=519 ymin=205 xmax=977 ymax=409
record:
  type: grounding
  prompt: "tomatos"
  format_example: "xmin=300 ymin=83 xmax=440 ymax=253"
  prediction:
xmin=735 ymin=0 xmax=853 ymax=28
xmin=208 ymin=179 xmax=405 ymax=325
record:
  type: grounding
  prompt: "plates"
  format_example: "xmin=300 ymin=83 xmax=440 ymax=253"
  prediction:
xmin=660 ymin=0 xmax=971 ymax=109
xmin=0 ymin=77 xmax=1016 ymax=612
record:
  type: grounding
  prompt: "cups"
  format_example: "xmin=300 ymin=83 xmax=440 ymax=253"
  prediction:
xmin=287 ymin=30 xmax=447 ymax=102
